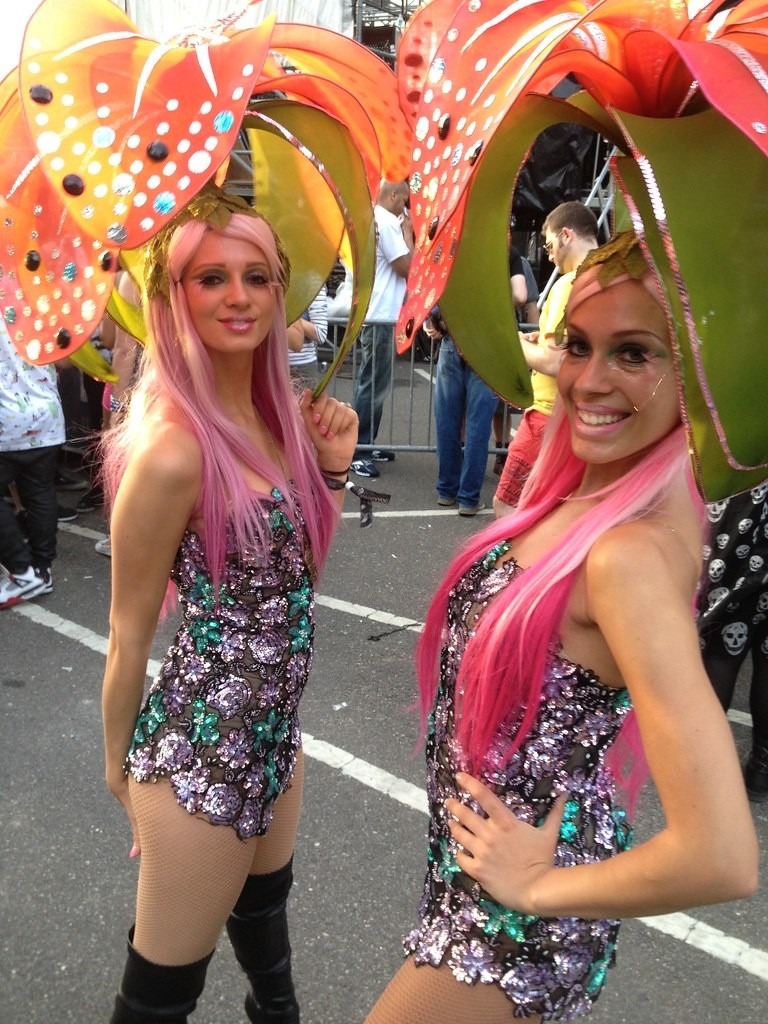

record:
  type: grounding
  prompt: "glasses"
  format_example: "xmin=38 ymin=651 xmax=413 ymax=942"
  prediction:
xmin=543 ymin=229 xmax=573 ymax=252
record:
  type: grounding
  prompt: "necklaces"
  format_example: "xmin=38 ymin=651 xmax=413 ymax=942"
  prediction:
xmin=263 ymin=422 xmax=288 ymax=484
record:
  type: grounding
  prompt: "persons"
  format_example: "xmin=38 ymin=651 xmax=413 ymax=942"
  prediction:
xmin=362 ymin=202 xmax=768 ymax=1024
xmin=101 ymin=178 xmax=359 ymax=1024
xmin=0 ymin=251 xmax=145 ymax=608
xmin=348 ymin=181 xmax=415 ymax=477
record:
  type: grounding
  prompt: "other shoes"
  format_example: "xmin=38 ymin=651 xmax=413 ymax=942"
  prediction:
xmin=745 ymin=741 xmax=768 ymax=801
xmin=494 ymin=442 xmax=511 ymax=475
xmin=77 ymin=488 xmax=108 ymax=512
xmin=95 ymin=539 xmax=111 ymax=555
xmin=437 ymin=495 xmax=456 ymax=506
xmin=54 ymin=475 xmax=89 ymax=491
xmin=458 ymin=499 xmax=486 ymax=516
xmin=58 ymin=504 xmax=79 ymax=521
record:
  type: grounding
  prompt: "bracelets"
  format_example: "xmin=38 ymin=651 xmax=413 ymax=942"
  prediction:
xmin=110 ymin=394 xmax=126 ymax=413
xmin=318 ymin=467 xmax=390 ymax=528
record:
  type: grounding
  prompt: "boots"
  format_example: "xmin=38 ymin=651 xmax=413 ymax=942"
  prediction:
xmin=226 ymin=851 xmax=300 ymax=1024
xmin=109 ymin=923 xmax=216 ymax=1024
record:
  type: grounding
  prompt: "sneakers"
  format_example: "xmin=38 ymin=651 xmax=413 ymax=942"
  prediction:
xmin=371 ymin=449 xmax=395 ymax=463
xmin=0 ymin=566 xmax=46 ymax=611
xmin=350 ymin=456 xmax=380 ymax=477
xmin=35 ymin=566 xmax=54 ymax=594
